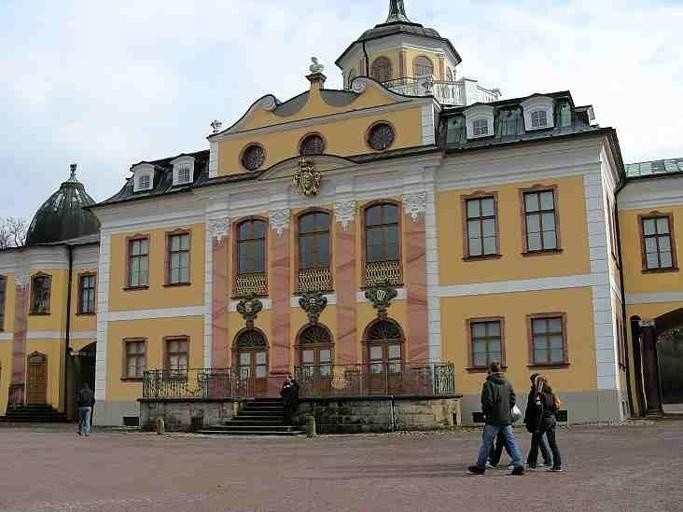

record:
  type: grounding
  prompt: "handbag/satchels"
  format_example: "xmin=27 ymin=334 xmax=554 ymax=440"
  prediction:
xmin=510 ymin=403 xmax=522 ymax=423
xmin=553 ymin=395 xmax=562 ymax=414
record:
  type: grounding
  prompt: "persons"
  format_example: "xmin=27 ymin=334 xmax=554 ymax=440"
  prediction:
xmin=484 ymin=429 xmax=515 ymax=469
xmin=466 ymin=361 xmax=524 ymax=475
xmin=279 ymin=373 xmax=299 ymax=425
xmin=528 ymin=374 xmax=552 ymax=466
xmin=522 ymin=373 xmax=562 ymax=473
xmin=308 ymin=56 xmax=324 ymax=73
xmin=73 ymin=382 xmax=94 ymax=436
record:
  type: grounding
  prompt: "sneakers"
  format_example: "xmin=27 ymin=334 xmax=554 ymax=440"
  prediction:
xmin=511 ymin=466 xmax=525 ymax=475
xmin=485 ymin=459 xmax=550 ymax=471
xmin=467 ymin=465 xmax=486 ymax=475
xmin=547 ymin=464 xmax=563 ymax=472
